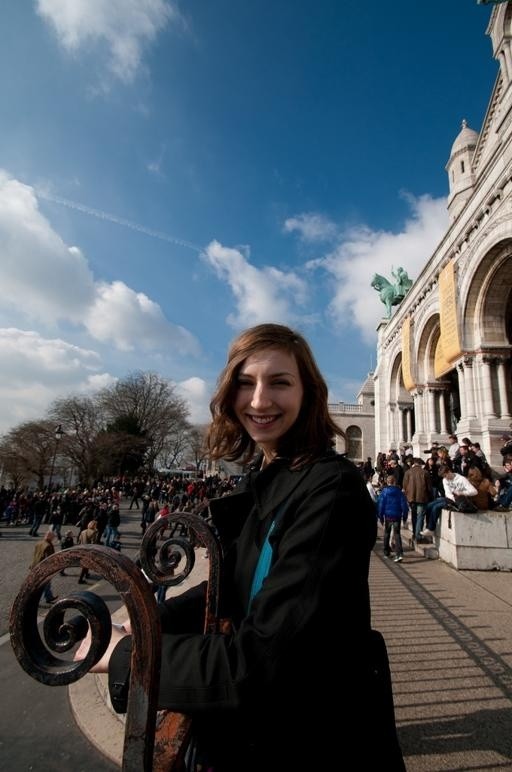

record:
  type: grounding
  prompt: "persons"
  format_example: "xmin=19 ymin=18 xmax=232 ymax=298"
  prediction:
xmin=71 ymin=320 xmax=404 ymax=772
xmin=1 ymin=468 xmax=244 ymax=605
xmin=353 ymin=433 xmax=511 ymax=565
xmin=391 ymin=265 xmax=409 ymax=299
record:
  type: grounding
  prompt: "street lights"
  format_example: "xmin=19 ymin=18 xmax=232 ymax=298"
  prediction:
xmin=47 ymin=421 xmax=64 ymax=495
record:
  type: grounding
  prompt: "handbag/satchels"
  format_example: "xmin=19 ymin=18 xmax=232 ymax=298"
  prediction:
xmin=195 ymin=623 xmax=408 ymax=772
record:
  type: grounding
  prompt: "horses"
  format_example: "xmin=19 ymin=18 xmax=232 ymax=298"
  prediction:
xmin=371 ymin=272 xmax=405 ymax=320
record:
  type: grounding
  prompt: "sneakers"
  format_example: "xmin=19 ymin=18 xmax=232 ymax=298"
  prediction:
xmin=384 ymin=551 xmax=404 ymax=563
xmin=419 ymin=528 xmax=435 ymax=537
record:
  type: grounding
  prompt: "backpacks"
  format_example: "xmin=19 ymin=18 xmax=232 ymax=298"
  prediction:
xmin=446 ymin=491 xmax=478 ymax=513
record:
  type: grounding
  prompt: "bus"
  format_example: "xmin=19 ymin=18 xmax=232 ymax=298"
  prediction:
xmin=159 ymin=468 xmax=203 ymax=482
